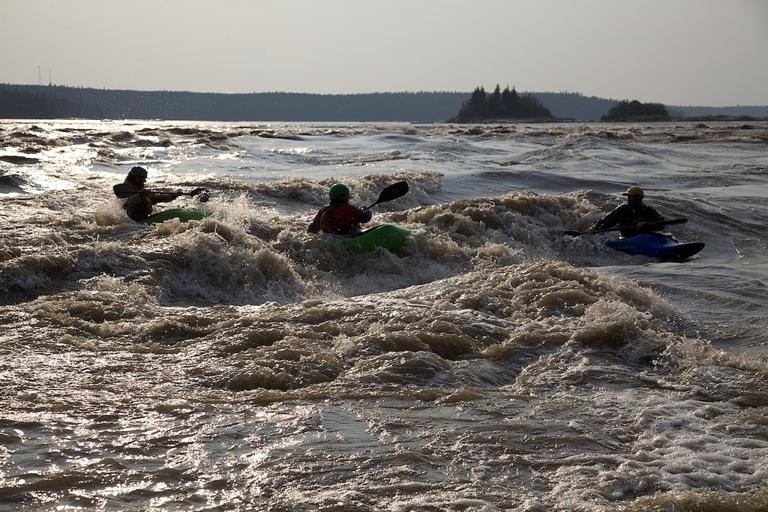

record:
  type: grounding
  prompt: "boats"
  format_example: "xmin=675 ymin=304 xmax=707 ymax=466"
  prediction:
xmin=138 ymin=207 xmax=218 ymax=225
xmin=602 ymin=230 xmax=706 ymax=262
xmin=326 ymin=222 xmax=414 ymax=257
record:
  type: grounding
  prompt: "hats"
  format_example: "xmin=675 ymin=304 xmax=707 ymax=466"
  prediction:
xmin=623 ymin=186 xmax=644 ymax=198
xmin=329 ymin=183 xmax=349 ymax=199
xmin=128 ymin=167 xmax=147 ymax=176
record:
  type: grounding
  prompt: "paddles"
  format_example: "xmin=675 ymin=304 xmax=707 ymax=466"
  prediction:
xmin=357 ymin=179 xmax=409 ymax=212
xmin=549 ymin=216 xmax=687 ymax=237
xmin=145 ymin=186 xmax=208 ymax=200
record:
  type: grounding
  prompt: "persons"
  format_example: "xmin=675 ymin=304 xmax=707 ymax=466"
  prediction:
xmin=586 ymin=186 xmax=665 ymax=238
xmin=307 ymin=183 xmax=372 ymax=236
xmin=113 ymin=167 xmax=184 ymax=220
